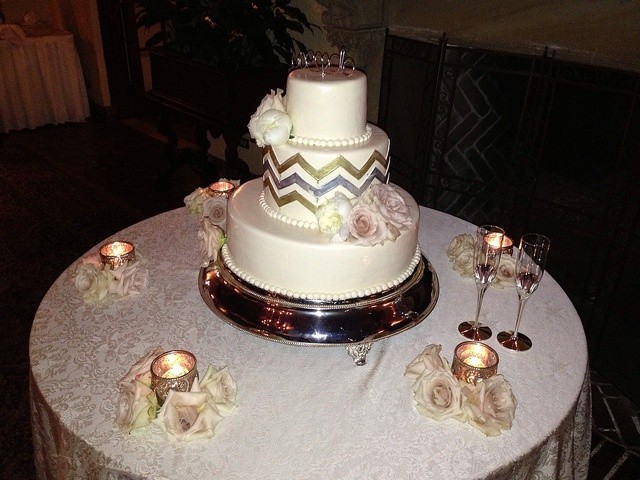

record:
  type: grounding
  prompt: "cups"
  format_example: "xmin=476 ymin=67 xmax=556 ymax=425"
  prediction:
xmin=450 ymin=341 xmax=499 ymax=386
xmin=482 ymin=231 xmax=514 ymax=259
xmin=99 ymin=240 xmax=136 ymax=280
xmin=149 ymin=350 xmax=198 ymax=401
xmin=208 ymin=181 xmax=235 ymax=198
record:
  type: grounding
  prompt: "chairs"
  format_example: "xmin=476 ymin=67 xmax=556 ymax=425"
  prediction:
xmin=0 ymin=25 xmax=49 ymax=132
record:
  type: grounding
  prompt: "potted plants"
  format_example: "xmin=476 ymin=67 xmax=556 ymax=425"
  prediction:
xmin=131 ymin=0 xmax=323 ymax=123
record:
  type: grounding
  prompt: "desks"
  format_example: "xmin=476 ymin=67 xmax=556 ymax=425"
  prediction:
xmin=145 ymin=88 xmax=258 ymax=182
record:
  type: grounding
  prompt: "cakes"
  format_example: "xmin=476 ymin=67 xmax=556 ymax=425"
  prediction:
xmin=227 ymin=68 xmax=421 ymax=302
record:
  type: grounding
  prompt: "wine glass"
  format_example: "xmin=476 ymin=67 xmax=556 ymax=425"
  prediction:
xmin=498 ymin=234 xmax=550 ymax=352
xmin=457 ymin=225 xmax=504 ymax=341
